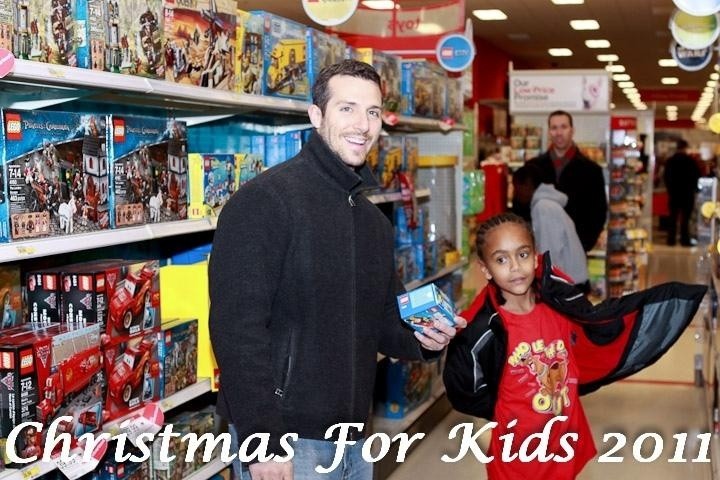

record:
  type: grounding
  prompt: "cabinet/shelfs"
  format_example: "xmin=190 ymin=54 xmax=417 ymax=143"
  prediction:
xmin=503 ymin=109 xmax=650 ymax=306
xmin=688 ymin=177 xmax=720 ymax=480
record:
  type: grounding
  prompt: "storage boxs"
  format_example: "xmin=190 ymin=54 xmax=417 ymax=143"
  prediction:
xmin=0 ymin=1 xmax=470 ymax=479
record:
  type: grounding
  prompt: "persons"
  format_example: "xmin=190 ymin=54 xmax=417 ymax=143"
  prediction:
xmin=442 ymin=210 xmax=708 ymax=480
xmin=512 ymin=166 xmax=590 ymax=289
xmin=526 ymin=111 xmax=609 ymax=253
xmin=207 ymin=58 xmax=467 ymax=479
xmin=662 ymin=139 xmax=702 ymax=247
